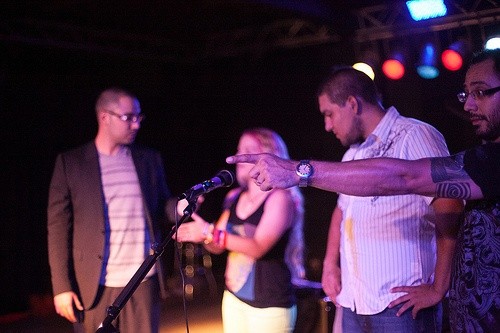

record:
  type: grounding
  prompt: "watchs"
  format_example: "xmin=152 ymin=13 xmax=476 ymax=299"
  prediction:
xmin=296 ymin=160 xmax=313 ymax=188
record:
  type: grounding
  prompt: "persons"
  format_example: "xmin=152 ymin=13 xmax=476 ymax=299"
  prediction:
xmin=47 ymin=89 xmax=204 ymax=333
xmin=317 ymin=68 xmax=462 ymax=333
xmin=227 ymin=48 xmax=500 ymax=333
xmin=172 ymin=129 xmax=305 ymax=333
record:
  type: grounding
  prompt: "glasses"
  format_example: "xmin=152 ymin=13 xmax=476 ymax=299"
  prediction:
xmin=104 ymin=109 xmax=144 ymax=124
xmin=456 ymin=86 xmax=500 ymax=102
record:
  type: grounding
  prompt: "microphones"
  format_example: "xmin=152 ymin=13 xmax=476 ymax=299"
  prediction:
xmin=177 ymin=169 xmax=236 ymax=200
